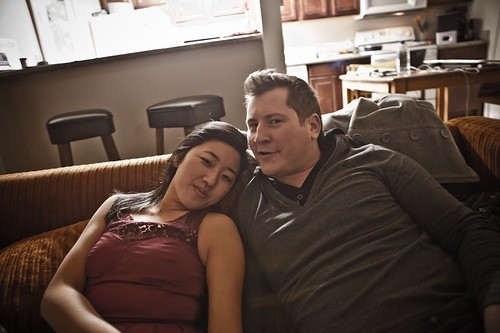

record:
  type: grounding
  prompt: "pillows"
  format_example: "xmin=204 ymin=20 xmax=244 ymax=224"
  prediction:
xmin=0 ymin=218 xmax=91 ymax=333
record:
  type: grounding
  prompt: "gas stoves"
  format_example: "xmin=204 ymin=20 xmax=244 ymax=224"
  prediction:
xmin=355 ymin=25 xmax=437 ymax=55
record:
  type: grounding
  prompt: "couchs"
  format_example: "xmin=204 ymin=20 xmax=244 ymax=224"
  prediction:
xmin=0 ymin=115 xmax=500 ymax=333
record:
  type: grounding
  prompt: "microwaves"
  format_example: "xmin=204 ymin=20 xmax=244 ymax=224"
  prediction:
xmin=353 ymin=0 xmax=427 ymax=21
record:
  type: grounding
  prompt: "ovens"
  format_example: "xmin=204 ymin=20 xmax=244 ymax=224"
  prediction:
xmin=371 ymin=50 xmax=437 ymax=110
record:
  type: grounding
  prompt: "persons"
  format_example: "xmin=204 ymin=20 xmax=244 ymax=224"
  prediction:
xmin=231 ymin=68 xmax=500 ymax=333
xmin=40 ymin=121 xmax=248 ymax=333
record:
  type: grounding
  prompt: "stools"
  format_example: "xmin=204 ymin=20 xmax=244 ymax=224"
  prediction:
xmin=146 ymin=94 xmax=225 ymax=156
xmin=46 ymin=108 xmax=122 ymax=168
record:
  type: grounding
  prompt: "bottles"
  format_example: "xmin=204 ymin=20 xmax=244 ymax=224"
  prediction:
xmin=397 ymin=40 xmax=410 ymax=76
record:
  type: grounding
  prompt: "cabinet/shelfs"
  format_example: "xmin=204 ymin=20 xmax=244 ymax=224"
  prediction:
xmin=280 ymin=0 xmax=360 ymax=22
xmin=305 ymin=56 xmax=371 ymax=115
xmin=437 ymin=43 xmax=487 ymax=60
xmin=338 ymin=64 xmax=499 ymax=123
